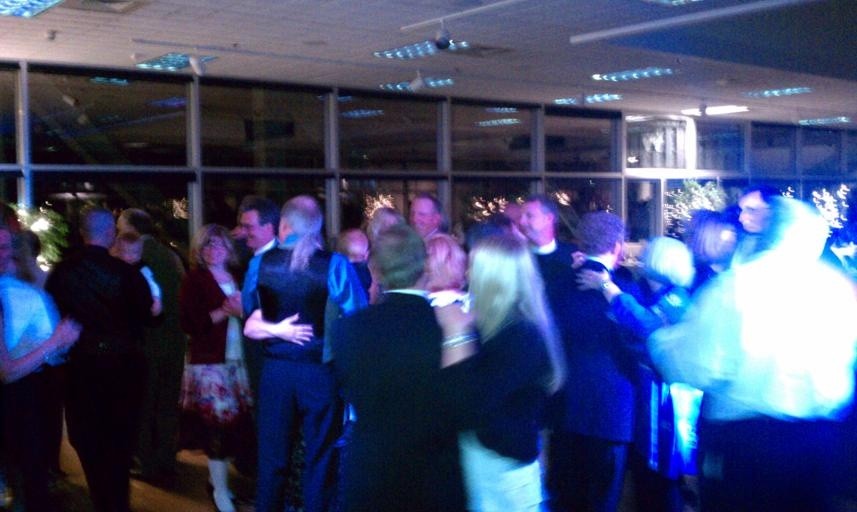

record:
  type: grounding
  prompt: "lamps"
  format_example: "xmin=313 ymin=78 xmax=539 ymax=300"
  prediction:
xmin=432 ymin=18 xmax=451 ymax=50
xmin=188 ymin=46 xmax=206 ymax=78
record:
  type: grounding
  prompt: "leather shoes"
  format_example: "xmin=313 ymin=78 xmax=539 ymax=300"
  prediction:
xmin=207 ymin=479 xmax=236 ymax=512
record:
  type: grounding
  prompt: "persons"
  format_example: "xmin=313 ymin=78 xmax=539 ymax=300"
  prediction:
xmin=0 ymin=188 xmax=857 ymax=511
